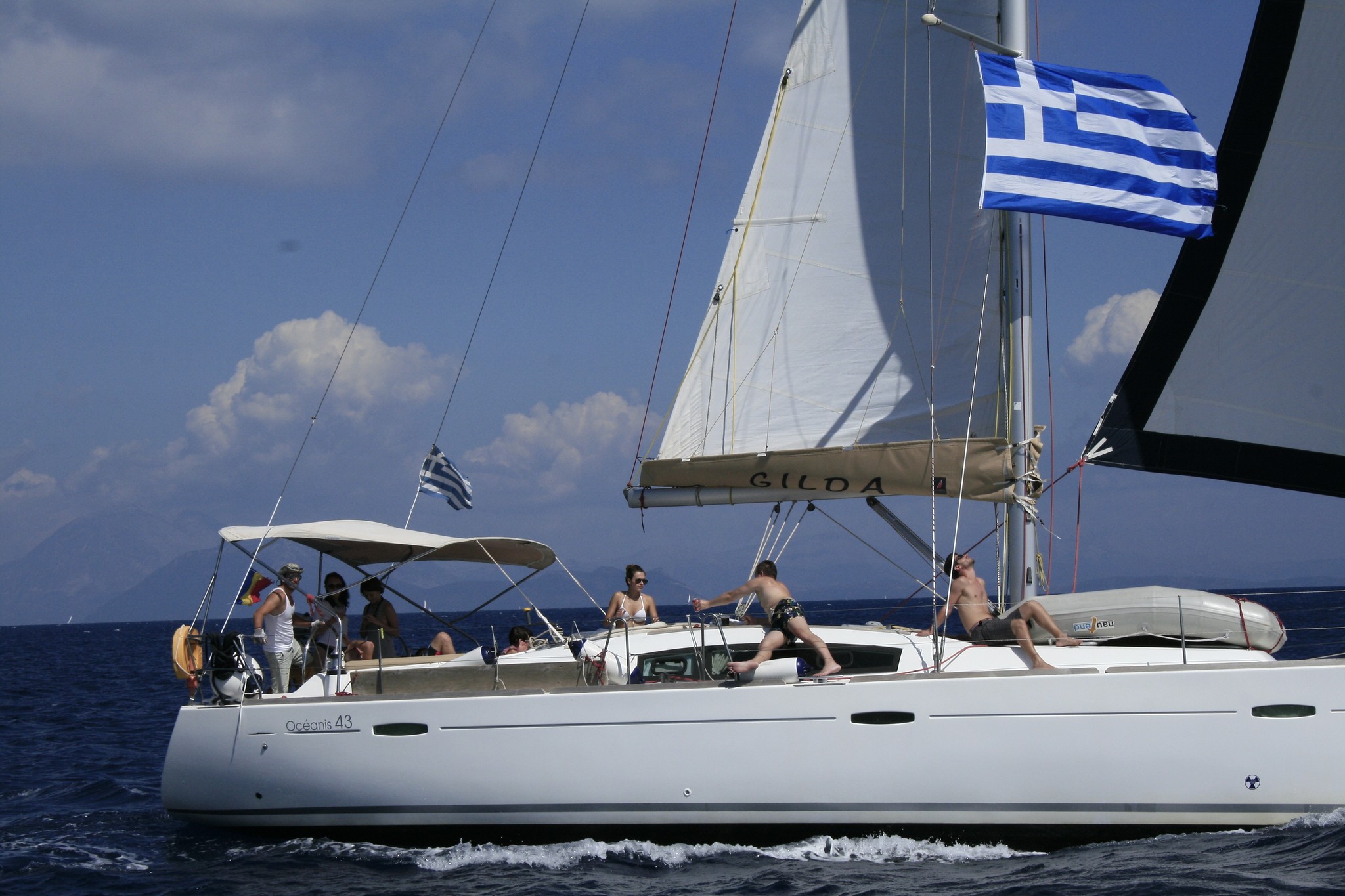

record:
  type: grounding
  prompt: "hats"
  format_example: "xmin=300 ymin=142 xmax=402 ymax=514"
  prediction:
xmin=278 ymin=563 xmax=304 ymax=580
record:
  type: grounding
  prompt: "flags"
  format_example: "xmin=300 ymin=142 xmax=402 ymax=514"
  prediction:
xmin=969 ymin=52 xmax=1217 ymax=240
xmin=417 ymin=445 xmax=473 ymax=511
xmin=238 ymin=569 xmax=273 ymax=605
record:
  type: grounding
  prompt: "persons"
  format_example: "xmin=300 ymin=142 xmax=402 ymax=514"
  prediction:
xmin=913 ymin=553 xmax=1082 ymax=670
xmin=694 ymin=560 xmax=842 ymax=676
xmin=251 ymin=564 xmax=454 ymax=693
xmin=502 ymin=625 xmax=533 ymax=656
xmin=602 ymin=564 xmax=661 ymax=630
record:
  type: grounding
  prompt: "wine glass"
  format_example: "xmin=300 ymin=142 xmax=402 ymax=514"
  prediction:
xmin=334 ymin=604 xmax=345 ymax=623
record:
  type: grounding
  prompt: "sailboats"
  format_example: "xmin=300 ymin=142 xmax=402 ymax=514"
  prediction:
xmin=159 ymin=1 xmax=1345 ymax=835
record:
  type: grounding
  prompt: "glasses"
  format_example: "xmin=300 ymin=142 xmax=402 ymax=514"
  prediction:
xmin=955 ymin=554 xmax=964 ymax=566
xmin=284 ymin=574 xmax=302 ymax=581
xmin=327 ymin=583 xmax=343 ymax=590
xmin=634 ymin=578 xmax=648 ymax=585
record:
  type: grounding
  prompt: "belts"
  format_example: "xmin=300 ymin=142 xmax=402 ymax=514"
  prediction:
xmin=969 ymin=619 xmax=993 ymax=639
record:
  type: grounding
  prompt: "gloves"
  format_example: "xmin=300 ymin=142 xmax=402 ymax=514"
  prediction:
xmin=253 ymin=628 xmax=268 ymax=645
xmin=311 ymin=619 xmax=325 ymax=630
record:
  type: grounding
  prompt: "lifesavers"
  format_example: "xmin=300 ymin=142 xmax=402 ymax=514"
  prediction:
xmin=173 ymin=624 xmax=201 ymax=680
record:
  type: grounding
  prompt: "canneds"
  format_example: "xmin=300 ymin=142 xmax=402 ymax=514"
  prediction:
xmin=692 ymin=598 xmax=701 ymax=612
